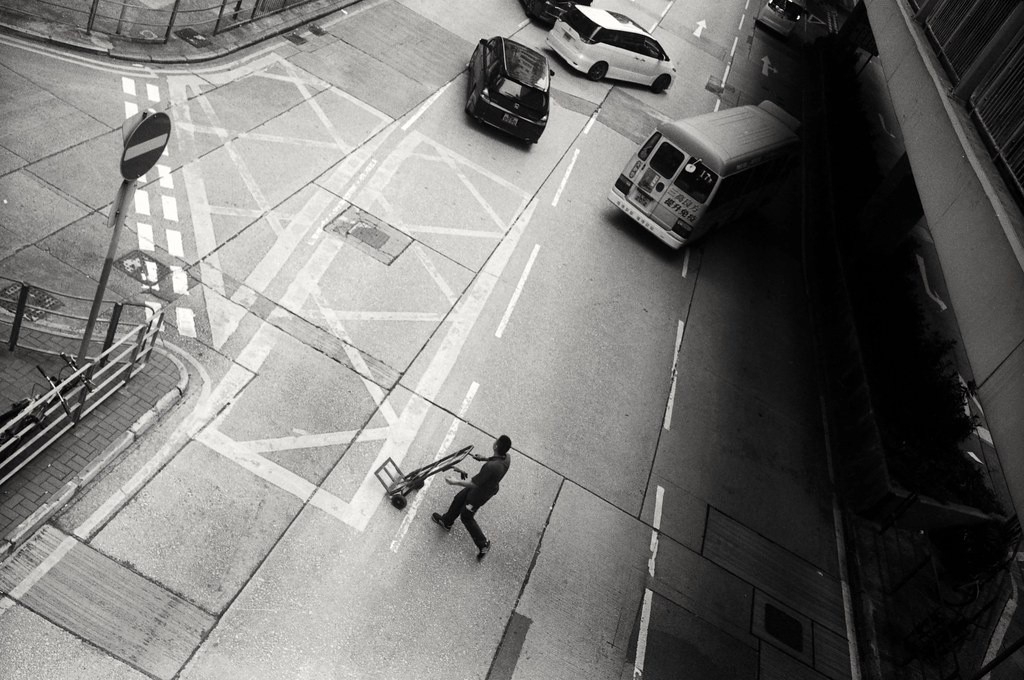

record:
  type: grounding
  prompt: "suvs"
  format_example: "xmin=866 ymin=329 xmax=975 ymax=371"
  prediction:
xmin=545 ymin=4 xmax=678 ymax=94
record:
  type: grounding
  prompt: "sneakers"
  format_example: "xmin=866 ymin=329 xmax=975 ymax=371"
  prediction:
xmin=432 ymin=513 xmax=452 ymax=531
xmin=478 ymin=539 xmax=490 ymax=559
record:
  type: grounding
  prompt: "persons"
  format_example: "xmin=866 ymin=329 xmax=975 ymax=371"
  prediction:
xmin=432 ymin=435 xmax=511 ymax=562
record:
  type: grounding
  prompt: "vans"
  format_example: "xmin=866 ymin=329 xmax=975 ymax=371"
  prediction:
xmin=606 ymin=100 xmax=805 ymax=250
xmin=755 ymin=0 xmax=805 ymax=41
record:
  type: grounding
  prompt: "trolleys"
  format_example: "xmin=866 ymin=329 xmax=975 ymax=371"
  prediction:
xmin=375 ymin=443 xmax=476 ymax=509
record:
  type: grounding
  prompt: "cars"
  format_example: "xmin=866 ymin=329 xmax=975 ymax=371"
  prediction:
xmin=519 ymin=0 xmax=594 ymax=30
xmin=464 ymin=36 xmax=555 ymax=145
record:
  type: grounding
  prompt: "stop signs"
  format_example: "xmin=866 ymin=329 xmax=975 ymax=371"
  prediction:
xmin=119 ymin=112 xmax=171 ymax=182
